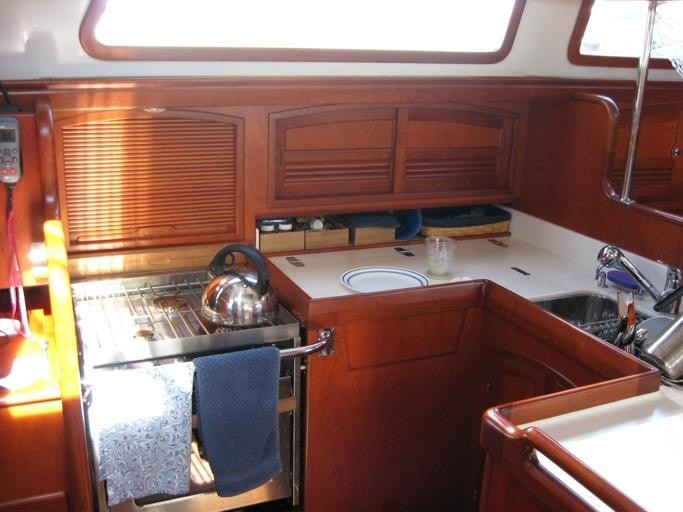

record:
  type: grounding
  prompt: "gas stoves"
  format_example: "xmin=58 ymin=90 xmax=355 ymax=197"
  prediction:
xmin=72 ymin=267 xmax=302 ymax=365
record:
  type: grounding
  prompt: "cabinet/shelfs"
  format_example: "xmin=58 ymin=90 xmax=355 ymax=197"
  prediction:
xmin=302 ymin=306 xmax=607 ymax=512
xmin=0 ymin=220 xmax=90 ymax=512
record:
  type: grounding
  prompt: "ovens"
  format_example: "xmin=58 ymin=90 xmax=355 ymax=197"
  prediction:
xmin=77 ymin=337 xmax=301 ymax=512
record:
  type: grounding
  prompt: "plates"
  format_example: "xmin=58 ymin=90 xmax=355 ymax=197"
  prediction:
xmin=340 ymin=266 xmax=431 ymax=294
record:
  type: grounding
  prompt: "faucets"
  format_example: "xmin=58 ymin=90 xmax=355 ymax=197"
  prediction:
xmin=599 ymin=245 xmax=683 ymax=314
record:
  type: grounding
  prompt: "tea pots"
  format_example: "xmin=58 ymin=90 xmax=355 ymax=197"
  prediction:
xmin=202 ymin=245 xmax=278 ymax=324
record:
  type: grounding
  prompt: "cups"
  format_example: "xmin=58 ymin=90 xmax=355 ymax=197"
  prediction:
xmin=425 ymin=237 xmax=457 ymax=276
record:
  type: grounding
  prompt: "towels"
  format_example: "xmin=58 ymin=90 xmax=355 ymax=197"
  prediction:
xmin=88 ymin=363 xmax=195 ymax=505
xmin=193 ymin=347 xmax=282 ymax=497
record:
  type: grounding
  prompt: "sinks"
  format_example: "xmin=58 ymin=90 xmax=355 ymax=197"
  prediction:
xmin=529 ymin=291 xmax=683 ymax=388
